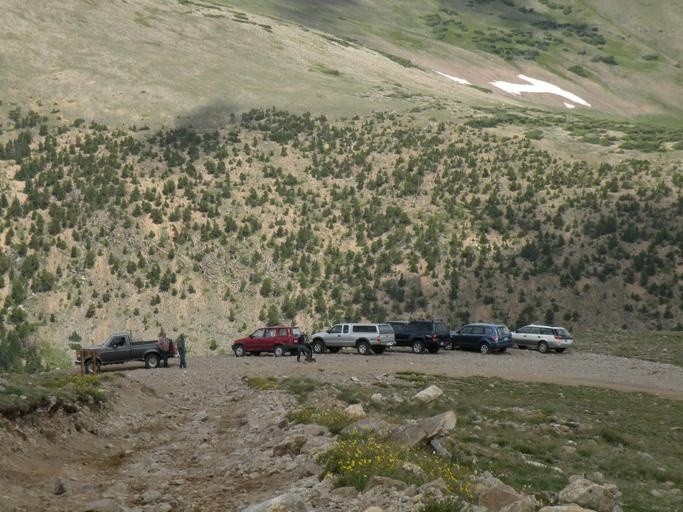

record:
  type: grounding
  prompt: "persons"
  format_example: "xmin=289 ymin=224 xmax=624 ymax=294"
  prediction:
xmin=303 ymin=341 xmax=316 ymax=363
xmin=296 ymin=332 xmax=307 ymax=362
xmin=176 ymin=333 xmax=186 ymax=368
xmin=158 ymin=332 xmax=170 ymax=368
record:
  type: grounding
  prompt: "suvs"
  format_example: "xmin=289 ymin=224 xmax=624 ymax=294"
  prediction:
xmin=382 ymin=319 xmax=453 ymax=354
xmin=308 ymin=321 xmax=396 ymax=356
xmin=510 ymin=323 xmax=576 ymax=355
xmin=231 ymin=325 xmax=304 ymax=358
xmin=440 ymin=322 xmax=515 ymax=355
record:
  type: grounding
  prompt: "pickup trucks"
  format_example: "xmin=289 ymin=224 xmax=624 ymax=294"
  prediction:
xmin=74 ymin=331 xmax=177 ymax=374
